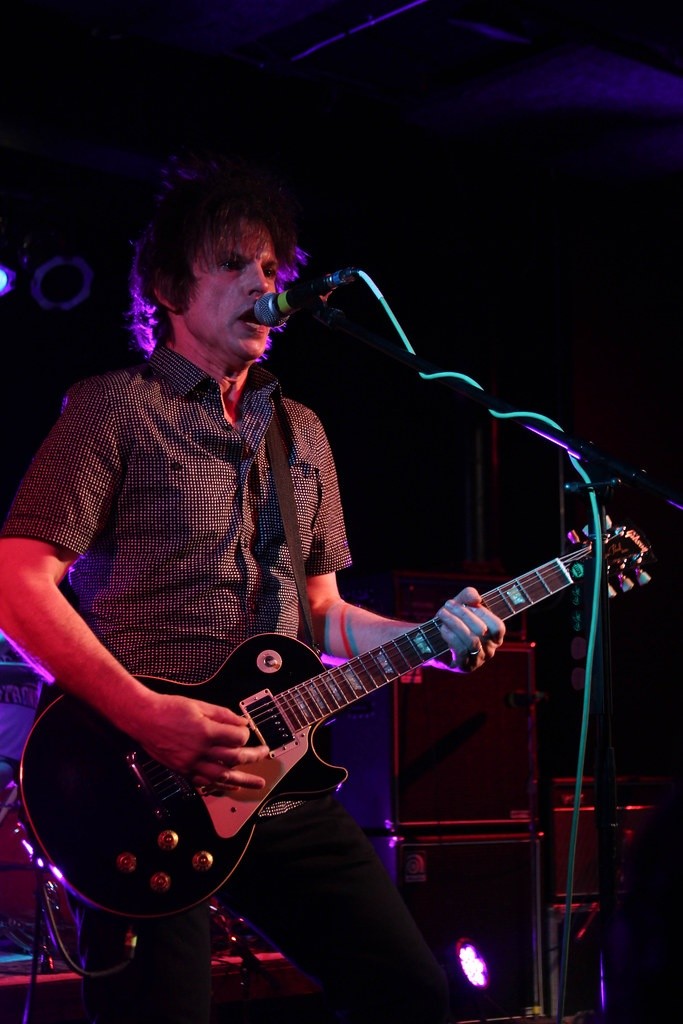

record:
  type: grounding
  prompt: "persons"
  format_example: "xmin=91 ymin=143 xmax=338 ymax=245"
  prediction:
xmin=0 ymin=181 xmax=507 ymax=1024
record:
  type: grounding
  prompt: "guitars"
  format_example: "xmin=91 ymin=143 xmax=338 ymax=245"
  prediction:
xmin=18 ymin=511 xmax=655 ymax=918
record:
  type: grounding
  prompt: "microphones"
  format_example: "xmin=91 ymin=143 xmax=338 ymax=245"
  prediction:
xmin=253 ymin=268 xmax=359 ymax=328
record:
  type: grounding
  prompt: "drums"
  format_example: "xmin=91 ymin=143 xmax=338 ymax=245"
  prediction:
xmin=0 ymin=775 xmax=82 ymax=975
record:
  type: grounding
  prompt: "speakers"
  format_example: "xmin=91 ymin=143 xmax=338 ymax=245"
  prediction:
xmin=327 ymin=646 xmax=673 ymax=1023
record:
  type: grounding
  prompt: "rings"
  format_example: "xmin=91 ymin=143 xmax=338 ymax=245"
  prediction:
xmin=471 ymin=645 xmax=483 ymax=656
